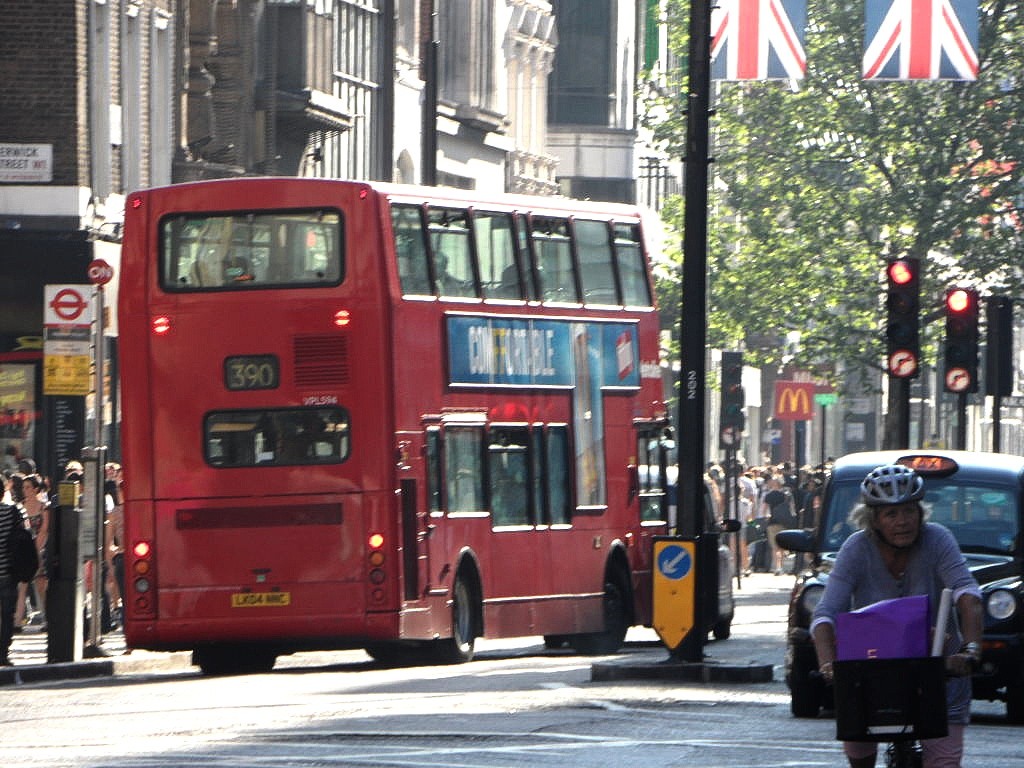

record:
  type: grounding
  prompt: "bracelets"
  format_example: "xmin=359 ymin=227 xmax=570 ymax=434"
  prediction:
xmin=963 ymin=642 xmax=982 ymax=653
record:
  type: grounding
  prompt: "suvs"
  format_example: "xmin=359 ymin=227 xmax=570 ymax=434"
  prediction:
xmin=773 ymin=449 xmax=1024 ymax=728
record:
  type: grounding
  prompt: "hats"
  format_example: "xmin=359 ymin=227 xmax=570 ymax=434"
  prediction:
xmin=64 ymin=461 xmax=82 ymax=470
xmin=105 ymin=462 xmax=121 ymax=471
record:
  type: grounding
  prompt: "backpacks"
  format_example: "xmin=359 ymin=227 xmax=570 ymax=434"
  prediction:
xmin=7 ymin=504 xmax=38 ymax=582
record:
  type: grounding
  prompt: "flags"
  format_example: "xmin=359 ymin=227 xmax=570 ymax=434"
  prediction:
xmin=861 ymin=0 xmax=980 ymax=82
xmin=709 ymin=0 xmax=809 ymax=82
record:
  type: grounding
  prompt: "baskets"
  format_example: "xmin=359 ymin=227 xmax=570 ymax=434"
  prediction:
xmin=830 ymin=654 xmax=949 ymax=743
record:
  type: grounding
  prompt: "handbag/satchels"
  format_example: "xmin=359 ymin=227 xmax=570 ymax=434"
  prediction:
xmin=836 ymin=592 xmax=932 ymax=660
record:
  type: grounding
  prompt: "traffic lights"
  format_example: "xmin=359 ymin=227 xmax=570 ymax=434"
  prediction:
xmin=885 ymin=256 xmax=921 ymax=378
xmin=719 ymin=351 xmax=746 ymax=450
xmin=942 ymin=286 xmax=980 ymax=394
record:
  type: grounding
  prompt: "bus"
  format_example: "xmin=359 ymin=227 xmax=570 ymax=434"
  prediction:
xmin=116 ymin=176 xmax=677 ymax=680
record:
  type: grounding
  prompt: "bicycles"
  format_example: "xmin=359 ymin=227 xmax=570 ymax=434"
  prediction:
xmin=810 ymin=654 xmax=996 ymax=768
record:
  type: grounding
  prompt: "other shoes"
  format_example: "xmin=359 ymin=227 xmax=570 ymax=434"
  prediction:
xmin=0 ymin=655 xmax=13 ymax=666
xmin=32 ymin=614 xmax=48 ymax=625
xmin=11 ymin=625 xmax=22 ymax=633
xmin=103 ymin=619 xmax=118 ymax=633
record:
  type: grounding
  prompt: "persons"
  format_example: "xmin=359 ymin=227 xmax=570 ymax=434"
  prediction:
xmin=0 ymin=455 xmax=138 ymax=666
xmin=701 ymin=459 xmax=835 ymax=577
xmin=810 ymin=462 xmax=985 ymax=767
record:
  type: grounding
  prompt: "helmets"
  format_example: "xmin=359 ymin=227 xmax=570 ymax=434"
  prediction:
xmin=861 ymin=464 xmax=925 ymax=505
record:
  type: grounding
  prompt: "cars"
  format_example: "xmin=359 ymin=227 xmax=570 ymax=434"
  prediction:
xmin=634 ymin=463 xmax=742 ymax=641
